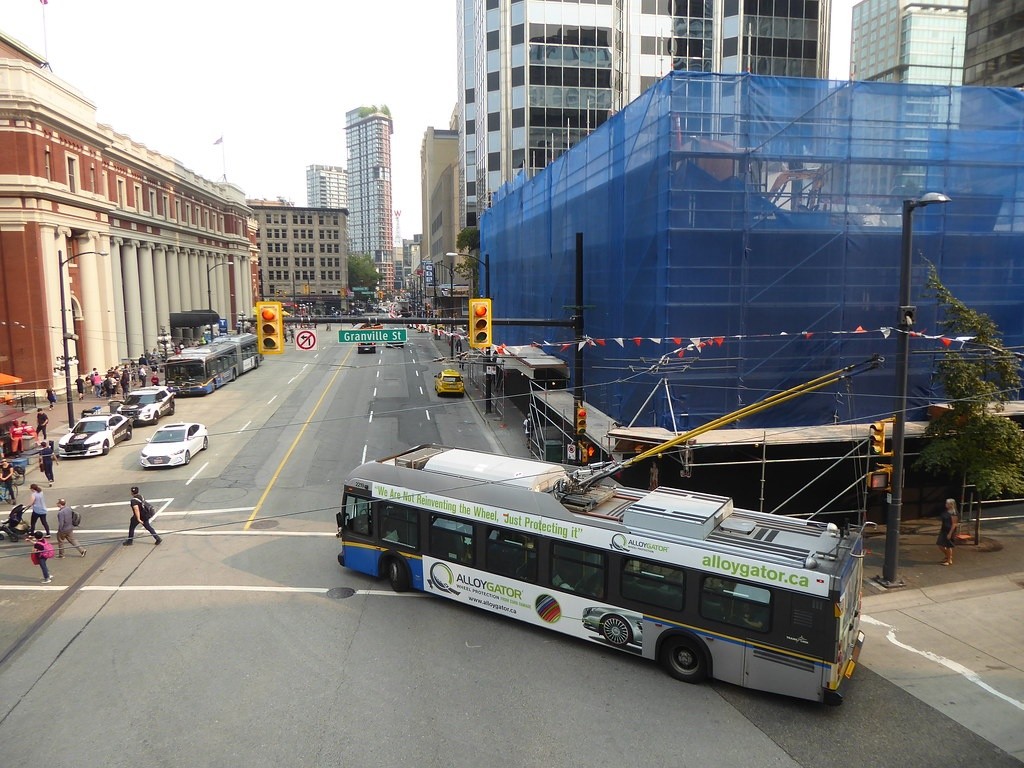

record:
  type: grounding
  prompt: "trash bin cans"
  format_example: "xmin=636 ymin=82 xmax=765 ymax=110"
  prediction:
xmin=109 ymin=401 xmax=120 ymax=414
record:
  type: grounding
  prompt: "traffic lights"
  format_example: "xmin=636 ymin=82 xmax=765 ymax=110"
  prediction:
xmin=469 ymin=299 xmax=492 ymax=348
xmin=586 ymin=444 xmax=595 ymax=457
xmin=578 ymin=448 xmax=588 ymax=463
xmin=577 ymin=407 xmax=587 ymax=434
xmin=869 ymin=420 xmax=885 ymax=456
xmin=868 ymin=471 xmax=891 ymax=488
xmin=257 ymin=301 xmax=284 ymax=354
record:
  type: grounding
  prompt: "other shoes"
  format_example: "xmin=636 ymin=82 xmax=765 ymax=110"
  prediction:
xmin=123 ymin=541 xmax=133 ymax=546
xmin=49 ymin=407 xmax=54 ymax=411
xmin=49 ymin=480 xmax=54 ymax=487
xmin=41 ymin=579 xmax=51 ymax=584
xmin=941 ymin=558 xmax=952 ymax=566
xmin=12 ymin=499 xmax=16 ymax=505
xmin=82 ymin=550 xmax=86 ymax=557
xmin=44 ymin=535 xmax=50 ymax=538
xmin=29 ymin=533 xmax=34 ymax=537
xmin=155 ymin=539 xmax=163 ymax=545
xmin=55 ymin=555 xmax=66 ymax=559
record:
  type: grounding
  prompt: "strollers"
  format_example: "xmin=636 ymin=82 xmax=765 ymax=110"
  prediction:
xmin=0 ymin=504 xmax=33 ymax=543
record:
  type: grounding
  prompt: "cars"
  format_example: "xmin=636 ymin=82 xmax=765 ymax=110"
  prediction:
xmin=434 ymin=369 xmax=465 ymax=398
xmin=357 ymin=343 xmax=376 ymax=354
xmin=352 ymin=324 xmax=384 ymax=329
xmin=116 ymin=386 xmax=175 ymax=428
xmin=300 ymin=323 xmax=318 ymax=329
xmin=386 ymin=342 xmax=404 ymax=349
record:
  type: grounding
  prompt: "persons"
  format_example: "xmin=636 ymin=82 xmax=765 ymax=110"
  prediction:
xmin=123 ymin=486 xmax=163 ymax=546
xmin=38 ymin=442 xmax=59 ymax=487
xmin=25 ymin=531 xmax=54 ymax=584
xmin=0 ymin=445 xmax=5 ymax=478
xmin=26 ymin=484 xmax=50 ymax=538
xmin=0 ymin=457 xmax=17 ymax=505
xmin=935 ymin=499 xmax=958 ymax=566
xmin=10 ymin=320 xmax=318 ymax=457
xmin=54 ymin=498 xmax=87 ymax=560
xmin=455 ymin=339 xmax=462 ymax=352
xmin=523 ymin=413 xmax=534 ymax=449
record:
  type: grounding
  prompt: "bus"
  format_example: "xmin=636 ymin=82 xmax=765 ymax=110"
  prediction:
xmin=160 ymin=332 xmax=264 ymax=398
xmin=334 ymin=352 xmax=885 ymax=707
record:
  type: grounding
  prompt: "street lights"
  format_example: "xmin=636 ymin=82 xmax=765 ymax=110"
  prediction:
xmin=426 ymin=263 xmax=455 ymax=360
xmin=881 ymin=192 xmax=953 ymax=581
xmin=307 ymin=274 xmax=322 ymax=315
xmin=236 ymin=310 xmax=248 ymax=333
xmin=57 ymin=250 xmax=110 ymax=429
xmin=206 ymin=262 xmax=234 ymax=342
xmin=157 ymin=324 xmax=172 ymax=360
xmin=446 ymin=252 xmax=492 ymax=415
xmin=408 ymin=273 xmax=422 ymax=318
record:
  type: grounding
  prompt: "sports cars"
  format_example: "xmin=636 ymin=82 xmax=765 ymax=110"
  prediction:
xmin=140 ymin=422 xmax=209 ymax=468
xmin=58 ymin=413 xmax=133 ymax=457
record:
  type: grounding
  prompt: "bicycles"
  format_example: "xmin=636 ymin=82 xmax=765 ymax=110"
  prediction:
xmin=0 ymin=466 xmax=26 ymax=505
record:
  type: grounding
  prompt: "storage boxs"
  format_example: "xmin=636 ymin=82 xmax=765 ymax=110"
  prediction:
xmin=12 ymin=436 xmax=51 ymax=468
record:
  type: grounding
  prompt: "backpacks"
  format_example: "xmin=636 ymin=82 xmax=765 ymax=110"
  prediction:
xmin=36 ymin=539 xmax=55 ymax=557
xmin=133 ymin=497 xmax=155 ymax=519
xmin=71 ymin=510 xmax=81 ymax=526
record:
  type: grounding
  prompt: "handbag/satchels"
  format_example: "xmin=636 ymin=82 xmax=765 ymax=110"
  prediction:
xmin=51 ymin=395 xmax=56 ymax=401
xmin=109 ymin=385 xmax=114 ymax=389
xmin=40 ymin=464 xmax=44 ymax=472
xmin=31 ymin=553 xmax=39 ymax=565
